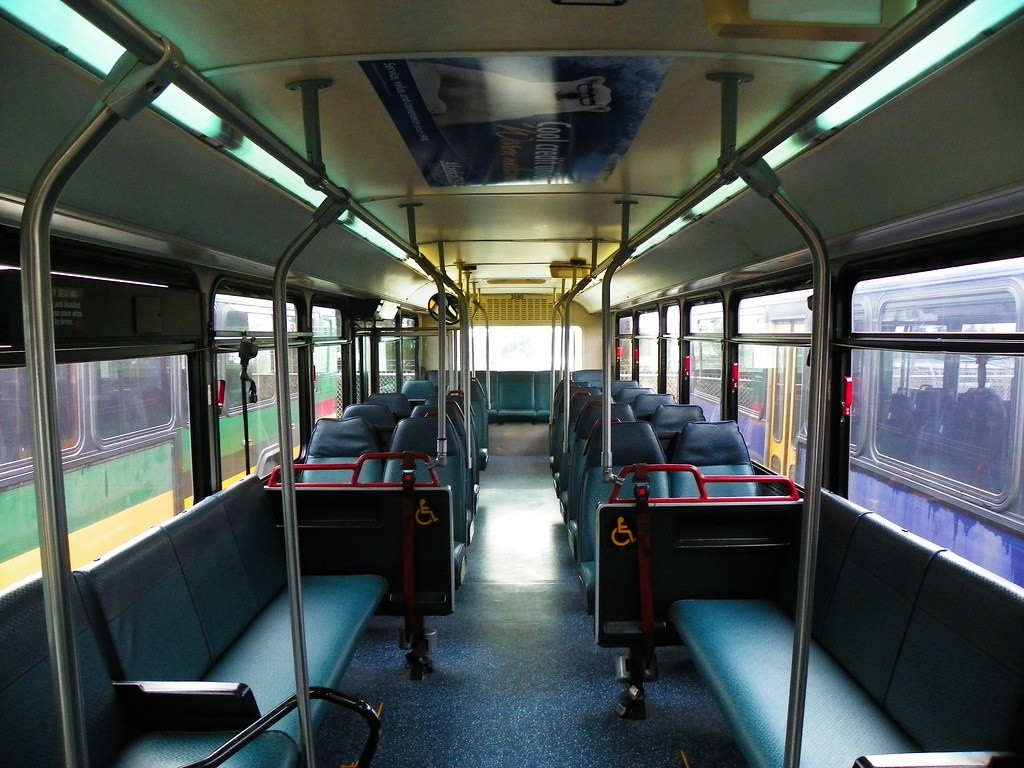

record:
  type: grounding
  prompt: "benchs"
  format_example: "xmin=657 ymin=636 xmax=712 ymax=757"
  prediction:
xmin=72 ymin=473 xmax=394 ymax=759
xmin=667 ymin=485 xmax=1024 ymax=768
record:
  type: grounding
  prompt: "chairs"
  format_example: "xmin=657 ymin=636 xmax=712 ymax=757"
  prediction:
xmin=112 ymin=388 xmax=149 ymax=431
xmin=94 ymin=396 xmax=127 ymax=438
xmin=296 ymin=369 xmax=761 ymax=618
xmin=0 ymin=571 xmax=303 ymax=768
xmin=879 ymin=383 xmax=1011 ymax=489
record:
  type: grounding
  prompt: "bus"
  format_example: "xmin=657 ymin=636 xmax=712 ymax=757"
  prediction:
xmin=0 ymin=294 xmax=342 ymax=598
xmin=620 ymin=256 xmax=1024 ymax=589
